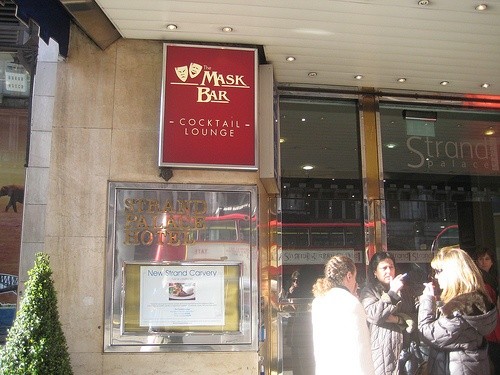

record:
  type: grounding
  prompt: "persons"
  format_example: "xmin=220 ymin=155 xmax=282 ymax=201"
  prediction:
xmin=287 ymin=268 xmax=316 ymax=375
xmin=419 ymin=247 xmax=500 ymax=375
xmin=362 ymin=251 xmax=415 ymax=375
xmin=312 ymin=255 xmax=374 ymax=375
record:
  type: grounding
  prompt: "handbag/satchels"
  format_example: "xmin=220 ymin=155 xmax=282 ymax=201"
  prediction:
xmin=399 ymin=341 xmax=439 ymax=375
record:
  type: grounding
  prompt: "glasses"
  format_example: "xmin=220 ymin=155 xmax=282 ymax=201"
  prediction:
xmin=435 ymin=269 xmax=443 ymax=274
